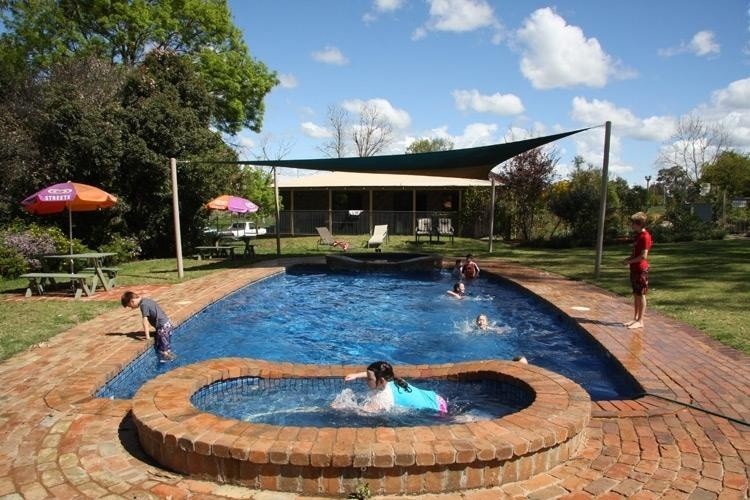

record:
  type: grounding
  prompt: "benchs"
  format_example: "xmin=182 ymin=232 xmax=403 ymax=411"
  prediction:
xmin=192 ymin=233 xmax=255 ymax=262
xmin=83 ymin=267 xmax=122 ymax=288
xmin=21 ymin=271 xmax=96 ymax=302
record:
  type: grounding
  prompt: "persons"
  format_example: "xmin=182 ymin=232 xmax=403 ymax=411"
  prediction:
xmin=623 ymin=211 xmax=651 ymax=328
xmin=121 ymin=292 xmax=177 ymax=365
xmin=462 ymin=314 xmax=507 ymax=335
xmin=331 ymin=361 xmax=448 ymax=417
xmin=512 ymin=355 xmax=528 ymax=364
xmin=446 ymin=254 xmax=480 ymax=298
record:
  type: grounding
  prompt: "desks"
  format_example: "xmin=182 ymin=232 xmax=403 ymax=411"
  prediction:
xmin=36 ymin=253 xmax=118 ymax=297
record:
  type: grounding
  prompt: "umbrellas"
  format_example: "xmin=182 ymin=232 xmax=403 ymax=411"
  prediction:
xmin=21 ymin=181 xmax=117 ymax=292
xmin=206 ymin=195 xmax=259 ymax=238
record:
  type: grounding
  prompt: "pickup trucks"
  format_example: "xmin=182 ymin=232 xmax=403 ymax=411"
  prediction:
xmin=217 ymin=221 xmax=268 ymax=240
xmin=198 ymin=226 xmax=218 ymax=237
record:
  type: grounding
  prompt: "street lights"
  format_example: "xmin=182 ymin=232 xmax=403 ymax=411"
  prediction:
xmin=644 ymin=174 xmax=651 ymax=212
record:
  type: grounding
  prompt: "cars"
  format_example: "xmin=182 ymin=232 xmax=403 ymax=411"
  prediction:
xmin=659 ymin=220 xmax=674 ymax=228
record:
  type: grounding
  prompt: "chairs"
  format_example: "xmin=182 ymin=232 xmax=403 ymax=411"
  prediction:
xmin=414 ymin=217 xmax=435 ymax=244
xmin=313 ymin=225 xmax=351 ymax=252
xmin=435 ymin=217 xmax=455 ymax=244
xmin=366 ymin=223 xmax=390 ymax=248
